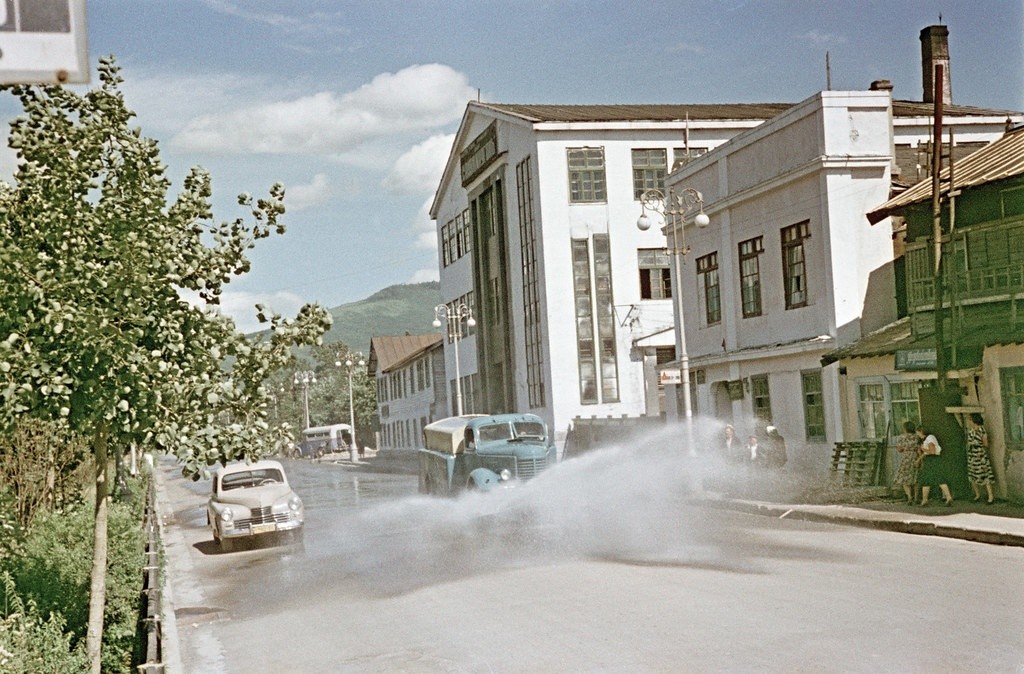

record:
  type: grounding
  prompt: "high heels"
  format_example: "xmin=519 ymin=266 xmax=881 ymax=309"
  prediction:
xmin=971 ymin=496 xmax=981 ymax=504
xmin=917 ymin=501 xmax=928 ymax=508
xmin=986 ymin=496 xmax=995 ymax=505
xmin=944 ymin=499 xmax=954 ymax=507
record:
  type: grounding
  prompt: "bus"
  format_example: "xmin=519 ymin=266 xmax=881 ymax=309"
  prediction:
xmin=294 ymin=424 xmax=354 ymax=460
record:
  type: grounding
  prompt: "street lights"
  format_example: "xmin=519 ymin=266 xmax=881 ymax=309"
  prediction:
xmin=431 ymin=303 xmax=477 ymax=417
xmin=266 ymin=381 xmax=286 ymax=421
xmin=334 ymin=347 xmax=366 ymax=462
xmin=637 ymin=182 xmax=711 ymax=494
xmin=294 ymin=370 xmax=317 ymax=430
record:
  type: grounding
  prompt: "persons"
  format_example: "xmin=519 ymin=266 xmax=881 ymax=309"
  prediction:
xmin=358 ymin=437 xmax=364 ymax=458
xmin=715 ymin=426 xmax=787 ymax=472
xmin=316 ymin=445 xmax=325 ymax=464
xmin=896 ymin=421 xmax=956 ymax=507
xmin=964 ymin=413 xmax=997 ymax=504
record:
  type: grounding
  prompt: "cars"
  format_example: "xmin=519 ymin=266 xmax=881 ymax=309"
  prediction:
xmin=206 ymin=459 xmax=307 ymax=551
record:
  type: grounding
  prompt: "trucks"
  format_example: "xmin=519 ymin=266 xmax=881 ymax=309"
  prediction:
xmin=417 ymin=410 xmax=564 ymax=510
